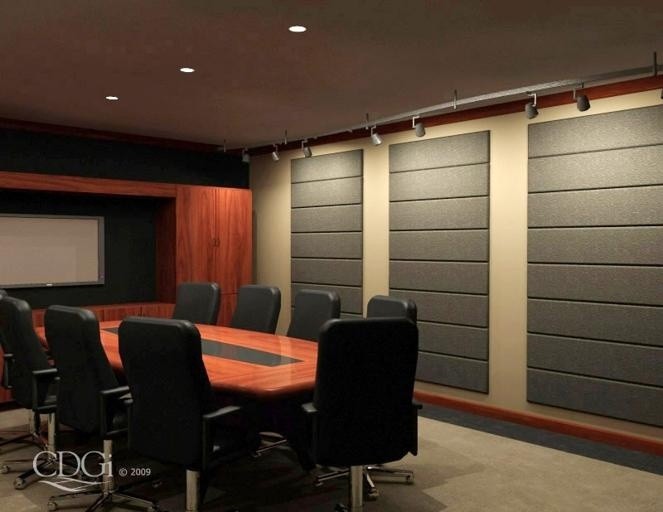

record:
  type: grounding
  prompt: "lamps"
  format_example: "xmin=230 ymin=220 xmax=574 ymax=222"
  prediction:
xmin=240 ymin=85 xmax=590 ymax=163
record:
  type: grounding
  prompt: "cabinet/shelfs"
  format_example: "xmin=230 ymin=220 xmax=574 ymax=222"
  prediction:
xmin=156 ymin=182 xmax=253 ymax=326
